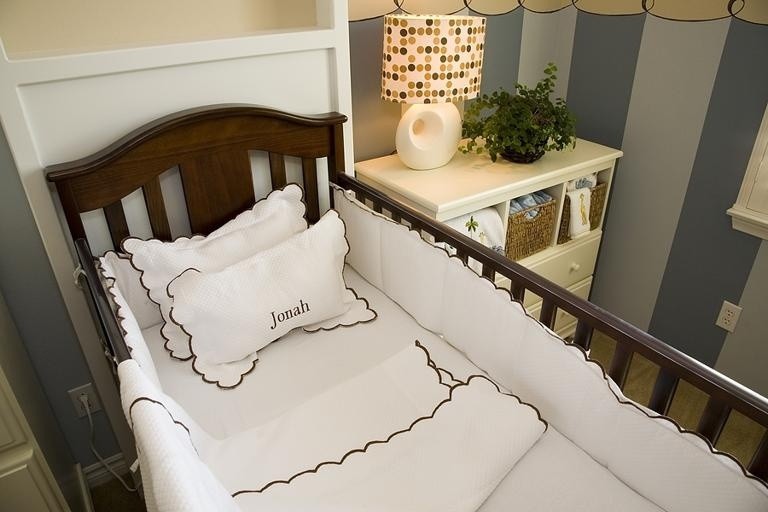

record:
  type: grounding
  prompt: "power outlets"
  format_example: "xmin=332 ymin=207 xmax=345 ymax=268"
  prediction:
xmin=66 ymin=382 xmax=103 ymax=420
xmin=715 ymin=298 xmax=743 ymax=335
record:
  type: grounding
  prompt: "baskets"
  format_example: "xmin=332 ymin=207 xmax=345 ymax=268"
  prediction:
xmin=505 ymin=197 xmax=556 ymax=262
xmin=557 ymin=180 xmax=608 ymax=246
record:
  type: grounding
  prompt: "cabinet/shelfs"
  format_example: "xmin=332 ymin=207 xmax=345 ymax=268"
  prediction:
xmin=352 ymin=127 xmax=624 ymax=340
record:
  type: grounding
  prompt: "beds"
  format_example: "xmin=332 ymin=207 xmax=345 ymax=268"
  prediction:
xmin=39 ymin=103 xmax=768 ymax=511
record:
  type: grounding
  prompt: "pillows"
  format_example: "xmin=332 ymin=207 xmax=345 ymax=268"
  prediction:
xmin=166 ymin=209 xmax=378 ymax=389
xmin=119 ymin=183 xmax=310 ymax=361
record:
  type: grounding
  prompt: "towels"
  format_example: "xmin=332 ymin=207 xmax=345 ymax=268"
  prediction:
xmin=442 ymin=206 xmax=506 ymax=254
xmin=565 ymin=186 xmax=591 ymax=240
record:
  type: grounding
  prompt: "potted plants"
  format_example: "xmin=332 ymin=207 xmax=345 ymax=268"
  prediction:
xmin=455 ymin=62 xmax=578 ymax=164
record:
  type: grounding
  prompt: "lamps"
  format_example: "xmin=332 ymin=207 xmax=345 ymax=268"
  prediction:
xmin=379 ymin=13 xmax=487 ymax=140
